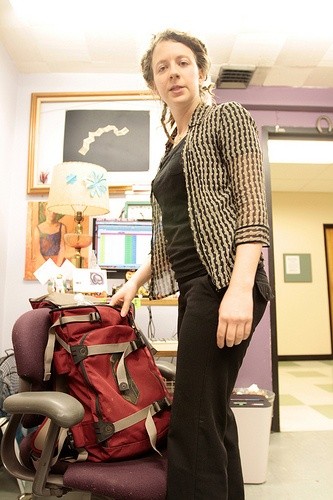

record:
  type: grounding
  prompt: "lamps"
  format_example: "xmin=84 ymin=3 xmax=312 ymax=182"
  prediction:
xmin=47 ymin=162 xmax=110 ymax=268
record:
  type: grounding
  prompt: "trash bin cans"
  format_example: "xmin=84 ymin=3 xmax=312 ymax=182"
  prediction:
xmin=230 ymin=384 xmax=275 ymax=419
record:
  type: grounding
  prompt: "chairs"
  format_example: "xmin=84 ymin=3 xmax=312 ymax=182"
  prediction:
xmin=1 ymin=307 xmax=168 ymax=500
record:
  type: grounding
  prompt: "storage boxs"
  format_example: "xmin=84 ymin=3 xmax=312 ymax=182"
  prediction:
xmin=93 ymin=216 xmax=152 ymax=278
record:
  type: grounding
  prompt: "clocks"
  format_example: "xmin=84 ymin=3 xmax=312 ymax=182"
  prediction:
xmin=125 ymin=201 xmax=152 ymax=219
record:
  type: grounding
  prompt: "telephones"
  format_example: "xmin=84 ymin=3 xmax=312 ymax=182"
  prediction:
xmin=125 ymin=271 xmax=150 ymax=298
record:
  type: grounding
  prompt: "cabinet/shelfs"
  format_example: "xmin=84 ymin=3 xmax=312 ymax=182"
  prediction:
xmin=133 ymin=298 xmax=179 ymax=357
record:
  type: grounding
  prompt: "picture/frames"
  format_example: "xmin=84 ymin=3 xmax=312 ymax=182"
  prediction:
xmin=26 ymin=91 xmax=177 ymax=194
xmin=24 ymin=201 xmax=90 ymax=281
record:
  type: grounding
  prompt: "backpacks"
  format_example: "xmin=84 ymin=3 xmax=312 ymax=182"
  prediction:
xmin=31 ymin=303 xmax=172 ymax=472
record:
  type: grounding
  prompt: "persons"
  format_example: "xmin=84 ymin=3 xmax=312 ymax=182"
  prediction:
xmin=109 ymin=28 xmax=275 ymax=500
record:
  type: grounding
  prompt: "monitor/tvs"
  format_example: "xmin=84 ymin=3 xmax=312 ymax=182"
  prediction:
xmin=93 ymin=217 xmax=152 ymax=271
